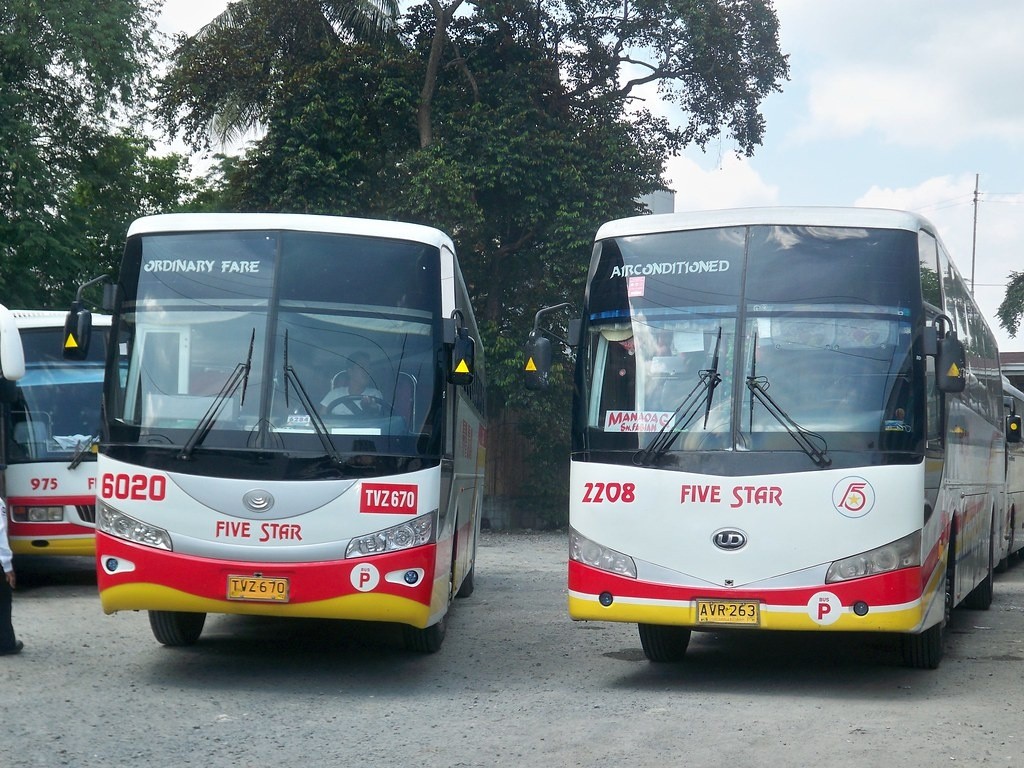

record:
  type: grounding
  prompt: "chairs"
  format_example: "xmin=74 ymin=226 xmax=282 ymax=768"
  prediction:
xmin=331 ymin=370 xmax=413 ymax=429
xmin=190 ymin=372 xmax=233 ymax=397
xmin=650 ymin=357 xmax=687 ymax=374
xmin=14 ymin=422 xmax=47 ymax=458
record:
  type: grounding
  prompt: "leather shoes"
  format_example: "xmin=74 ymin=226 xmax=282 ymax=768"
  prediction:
xmin=1 ymin=640 xmax=23 ymax=656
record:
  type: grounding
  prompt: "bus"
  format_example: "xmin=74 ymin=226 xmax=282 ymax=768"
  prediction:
xmin=522 ymin=206 xmax=1024 ymax=670
xmin=0 ymin=305 xmax=136 ymax=558
xmin=63 ymin=213 xmax=489 ymax=656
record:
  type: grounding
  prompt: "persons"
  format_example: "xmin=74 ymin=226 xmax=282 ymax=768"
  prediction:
xmin=316 ymin=351 xmax=387 ymax=420
xmin=0 ymin=497 xmax=24 ymax=656
